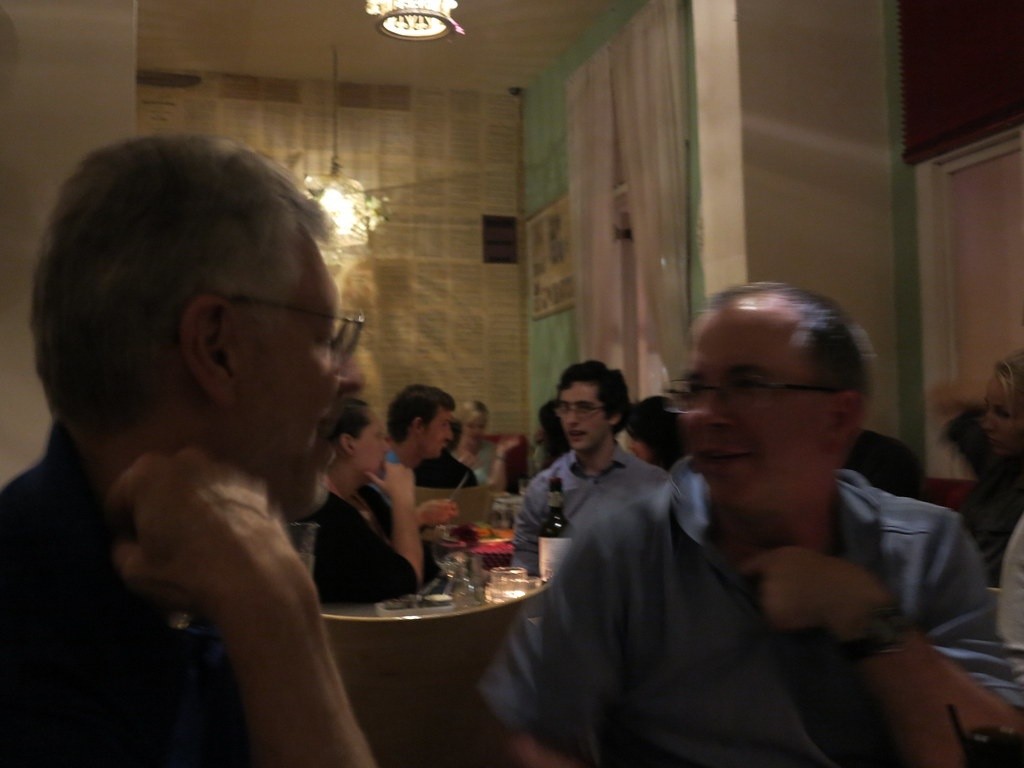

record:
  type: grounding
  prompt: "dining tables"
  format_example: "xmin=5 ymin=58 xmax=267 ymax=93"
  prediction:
xmin=430 ymin=524 xmax=520 ymax=571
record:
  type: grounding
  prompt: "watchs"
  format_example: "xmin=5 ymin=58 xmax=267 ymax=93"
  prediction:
xmin=830 ymin=594 xmax=921 ymax=660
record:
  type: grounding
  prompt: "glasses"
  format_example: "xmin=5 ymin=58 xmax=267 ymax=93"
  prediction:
xmin=549 ymin=401 xmax=606 ymax=418
xmin=663 ymin=368 xmax=860 ymax=416
xmin=225 ymin=291 xmax=366 ymax=370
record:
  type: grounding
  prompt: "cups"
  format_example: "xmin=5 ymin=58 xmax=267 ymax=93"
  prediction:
xmin=492 ymin=566 xmax=526 ymax=602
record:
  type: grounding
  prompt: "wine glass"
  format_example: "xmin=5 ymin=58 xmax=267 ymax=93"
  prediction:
xmin=434 ymin=520 xmax=470 ymax=604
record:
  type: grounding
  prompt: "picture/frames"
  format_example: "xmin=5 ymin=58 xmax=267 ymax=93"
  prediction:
xmin=523 ymin=192 xmax=582 ymax=323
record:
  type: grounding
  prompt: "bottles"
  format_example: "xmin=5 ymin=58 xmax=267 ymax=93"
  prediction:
xmin=539 ymin=477 xmax=571 ymax=584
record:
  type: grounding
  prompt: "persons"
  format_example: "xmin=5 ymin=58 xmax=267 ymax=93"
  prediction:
xmin=472 ymin=280 xmax=1024 ymax=768
xmin=509 ymin=357 xmax=669 ymax=579
xmin=623 ymin=395 xmax=688 ymax=470
xmin=927 ymin=348 xmax=1023 ymax=681
xmin=535 ymin=402 xmax=574 ymax=471
xmin=447 ymin=399 xmax=522 ymax=492
xmin=303 ymin=393 xmax=424 ymax=609
xmin=366 ymin=383 xmax=459 ymax=534
xmin=0 ymin=134 xmax=377 ymax=768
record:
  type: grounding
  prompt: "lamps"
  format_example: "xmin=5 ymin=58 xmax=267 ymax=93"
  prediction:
xmin=365 ymin=0 xmax=464 ymax=42
xmin=297 ymin=46 xmax=386 ymax=251
